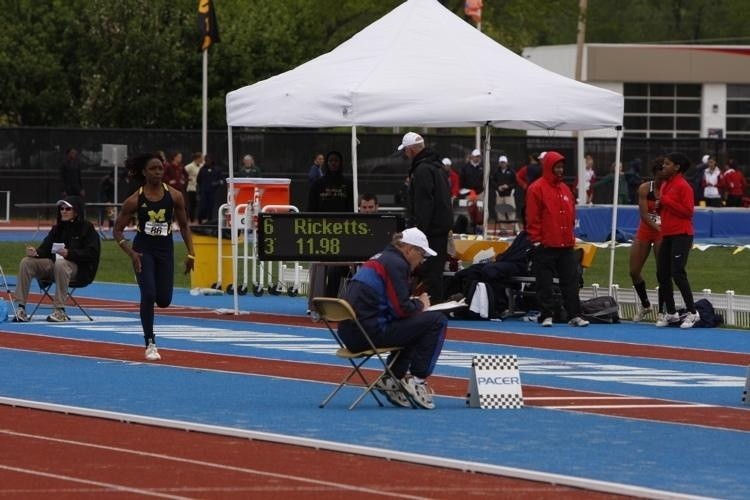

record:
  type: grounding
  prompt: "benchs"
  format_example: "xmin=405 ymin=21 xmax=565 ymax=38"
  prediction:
xmin=441 ymin=238 xmax=598 ymax=318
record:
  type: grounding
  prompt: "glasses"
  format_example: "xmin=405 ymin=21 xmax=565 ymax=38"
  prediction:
xmin=418 ymin=249 xmax=427 ymax=262
xmin=60 ymin=207 xmax=71 ymax=211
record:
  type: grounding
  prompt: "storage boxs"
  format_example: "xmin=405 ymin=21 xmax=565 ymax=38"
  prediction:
xmin=225 ymin=177 xmax=293 ymax=226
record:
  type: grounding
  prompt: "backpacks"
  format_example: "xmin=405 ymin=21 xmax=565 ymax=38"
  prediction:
xmin=552 ymin=296 xmax=621 ymax=324
xmin=668 ymin=299 xmax=724 ymax=327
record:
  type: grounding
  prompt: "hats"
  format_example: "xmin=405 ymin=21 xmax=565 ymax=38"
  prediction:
xmin=397 ymin=131 xmax=424 ymax=151
xmin=499 ymin=156 xmax=508 ymax=163
xmin=472 ymin=149 xmax=481 ymax=157
xmin=57 ymin=200 xmax=72 ymax=208
xmin=397 ymin=227 xmax=438 ymax=256
xmin=442 ymin=158 xmax=452 ymax=165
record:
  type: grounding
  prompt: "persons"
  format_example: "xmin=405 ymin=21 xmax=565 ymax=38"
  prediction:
xmin=627 ymin=158 xmax=665 ymax=326
xmin=398 ymin=133 xmax=455 ymax=302
xmin=337 ymin=227 xmax=446 ymax=410
xmin=653 ymin=151 xmax=700 ymax=329
xmin=13 ymin=195 xmax=99 ymax=323
xmin=324 ymin=192 xmax=393 ymax=299
xmin=113 ymin=152 xmax=196 ymax=361
xmin=57 ymin=149 xmax=749 ymax=230
xmin=446 ymin=205 xmax=537 ymax=322
xmin=526 ymin=151 xmax=593 ymax=327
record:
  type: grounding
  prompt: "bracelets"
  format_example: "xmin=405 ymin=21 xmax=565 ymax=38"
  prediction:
xmin=188 ymin=254 xmax=195 ymax=259
xmin=118 ymin=240 xmax=126 ymax=245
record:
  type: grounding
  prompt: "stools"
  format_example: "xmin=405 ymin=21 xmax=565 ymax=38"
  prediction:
xmin=27 ymin=273 xmax=94 ymax=323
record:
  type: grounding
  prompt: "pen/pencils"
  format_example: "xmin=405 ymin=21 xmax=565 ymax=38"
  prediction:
xmin=409 ymin=295 xmax=432 ymax=300
xmin=25 ymin=240 xmax=39 ymax=257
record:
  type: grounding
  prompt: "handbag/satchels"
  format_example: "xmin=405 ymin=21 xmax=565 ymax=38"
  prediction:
xmin=338 ymin=267 xmax=355 ymax=301
xmin=468 ymin=199 xmax=485 ymax=224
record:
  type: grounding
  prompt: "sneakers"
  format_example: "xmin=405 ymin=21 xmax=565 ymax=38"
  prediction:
xmin=634 ymin=302 xmax=654 ymax=322
xmin=145 ymin=343 xmax=161 ymax=361
xmin=542 ymin=317 xmax=553 ymax=327
xmin=400 ymin=376 xmax=436 ymax=409
xmin=568 ymin=317 xmax=589 ymax=327
xmin=529 ymin=312 xmax=542 ymax=321
xmin=47 ymin=309 xmax=68 ymax=322
xmin=374 ymin=375 xmax=412 ymax=408
xmin=12 ymin=307 xmax=28 ymax=321
xmin=655 ymin=311 xmax=681 ymax=327
xmin=656 ymin=310 xmax=667 ymax=322
xmin=680 ymin=310 xmax=701 ymax=328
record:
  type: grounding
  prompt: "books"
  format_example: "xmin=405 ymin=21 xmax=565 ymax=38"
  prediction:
xmin=422 ymin=297 xmax=467 ymax=311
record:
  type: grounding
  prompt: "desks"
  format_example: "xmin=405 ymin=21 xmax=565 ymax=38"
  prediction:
xmin=14 ymin=202 xmax=125 ymax=240
xmin=573 ymin=204 xmax=750 ymax=246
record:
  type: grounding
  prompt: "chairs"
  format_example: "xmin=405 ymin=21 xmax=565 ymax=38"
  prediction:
xmin=312 ymin=295 xmax=421 ymax=412
xmin=493 ymin=203 xmax=521 ymax=236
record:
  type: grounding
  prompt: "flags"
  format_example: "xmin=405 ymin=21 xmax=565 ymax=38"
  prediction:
xmin=464 ymin=0 xmax=484 ymax=24
xmin=195 ymin=0 xmax=222 ymax=55
